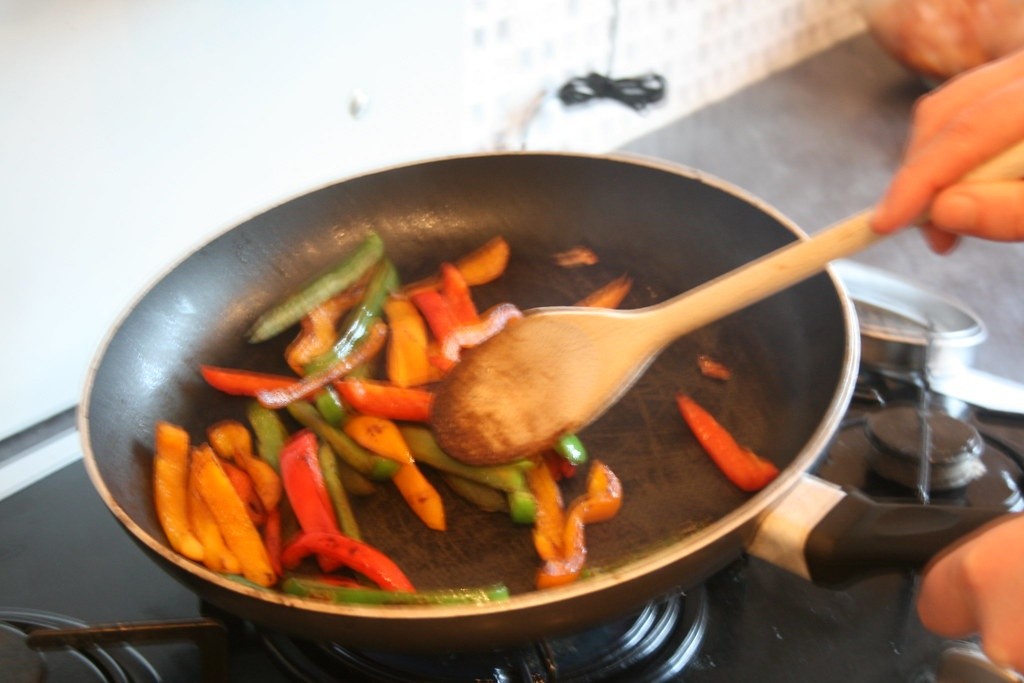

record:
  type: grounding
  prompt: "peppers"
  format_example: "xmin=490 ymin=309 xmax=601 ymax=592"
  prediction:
xmin=152 ymin=236 xmax=777 ymax=606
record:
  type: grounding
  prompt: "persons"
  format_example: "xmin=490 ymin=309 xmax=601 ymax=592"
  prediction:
xmin=869 ymin=47 xmax=1024 ymax=678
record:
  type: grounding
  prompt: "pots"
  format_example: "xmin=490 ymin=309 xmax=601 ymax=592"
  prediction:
xmin=77 ymin=147 xmax=1008 ymax=645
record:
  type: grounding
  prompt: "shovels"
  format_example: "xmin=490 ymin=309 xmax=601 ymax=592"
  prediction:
xmin=429 ymin=139 xmax=1024 ymax=466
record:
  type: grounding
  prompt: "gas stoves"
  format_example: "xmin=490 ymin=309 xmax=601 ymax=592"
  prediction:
xmin=0 ymin=247 xmax=1024 ymax=683
